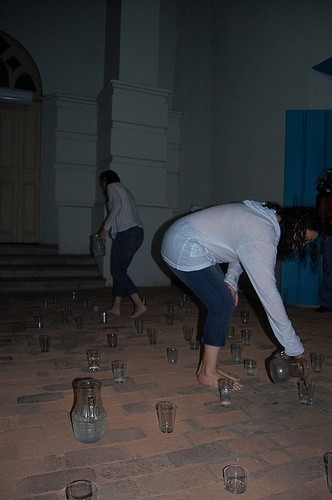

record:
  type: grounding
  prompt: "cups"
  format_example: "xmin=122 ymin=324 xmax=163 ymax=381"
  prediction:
xmin=167 ymin=348 xmax=178 ymax=364
xmin=241 ymin=329 xmax=252 ymax=346
xmin=135 ymin=296 xmax=157 ymax=345
xmin=297 ymin=379 xmax=312 ymax=405
xmin=323 ymin=452 xmax=332 ymax=492
xmin=111 ymin=360 xmax=127 ymax=383
xmin=156 ymin=401 xmax=177 ymax=433
xmin=65 ymin=480 xmax=98 ymax=500
xmin=242 ymin=311 xmax=249 ymax=323
xmin=107 ymin=333 xmax=118 ymax=348
xmin=223 ymin=465 xmax=250 ymax=494
xmin=86 ymin=349 xmax=100 ymax=370
xmin=166 ymin=294 xmax=192 ymax=326
xmin=182 ymin=326 xmax=200 ymax=350
xmin=218 ymin=378 xmax=234 ymax=408
xmin=35 ymin=290 xmax=107 ymax=352
xmin=244 ymin=359 xmax=257 ymax=376
xmin=310 ymin=351 xmax=325 ymax=372
xmin=230 ymin=342 xmax=243 ymax=364
xmin=228 ymin=327 xmax=235 ymax=339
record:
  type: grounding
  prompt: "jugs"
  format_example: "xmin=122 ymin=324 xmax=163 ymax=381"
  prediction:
xmin=269 ymin=350 xmax=309 ymax=385
xmin=72 ymin=378 xmax=106 ymax=443
xmin=89 ymin=233 xmax=106 ymax=259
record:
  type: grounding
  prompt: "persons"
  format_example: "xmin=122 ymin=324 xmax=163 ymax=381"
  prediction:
xmin=97 ymin=170 xmax=148 ymax=319
xmin=161 ymin=200 xmax=326 ymax=391
